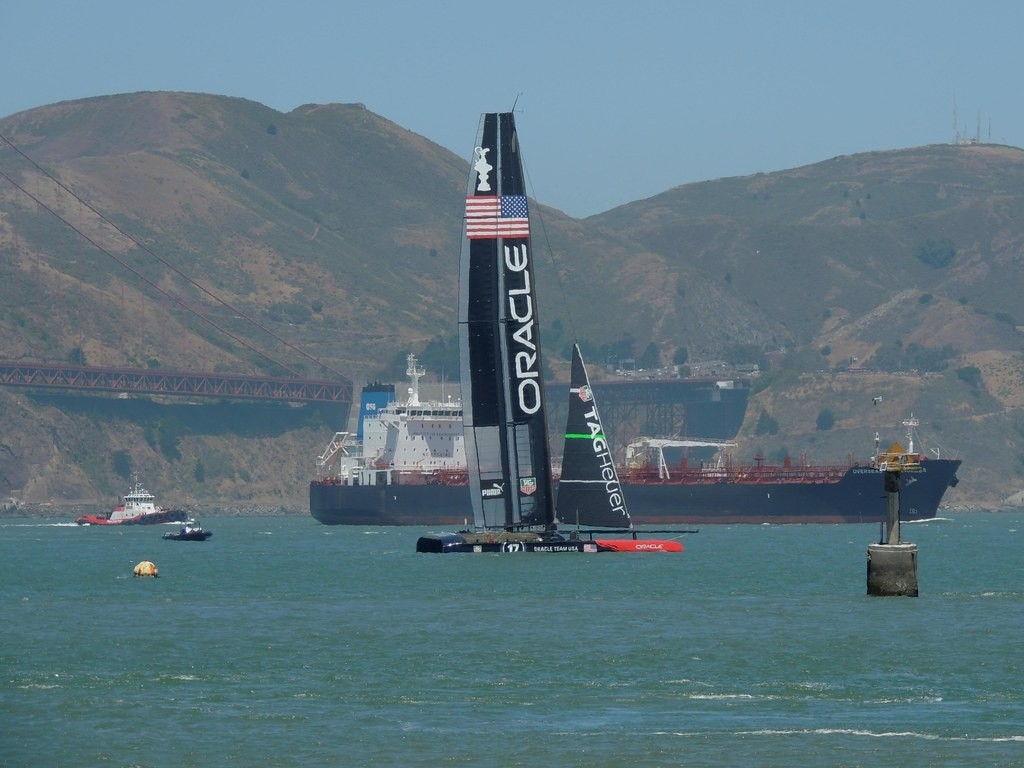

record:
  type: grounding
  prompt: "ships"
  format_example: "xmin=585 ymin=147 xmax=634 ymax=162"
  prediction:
xmin=310 ymin=430 xmax=963 ymax=525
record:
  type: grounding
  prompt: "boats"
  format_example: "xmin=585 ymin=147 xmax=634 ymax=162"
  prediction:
xmin=75 ymin=484 xmax=184 ymax=526
xmin=161 ymin=523 xmax=217 ymax=542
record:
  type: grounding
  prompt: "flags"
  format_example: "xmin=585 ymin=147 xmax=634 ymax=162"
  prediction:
xmin=466 ymin=195 xmax=530 ymax=239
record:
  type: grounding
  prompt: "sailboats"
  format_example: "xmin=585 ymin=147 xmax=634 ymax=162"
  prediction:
xmin=415 ymin=113 xmax=686 ymax=553
